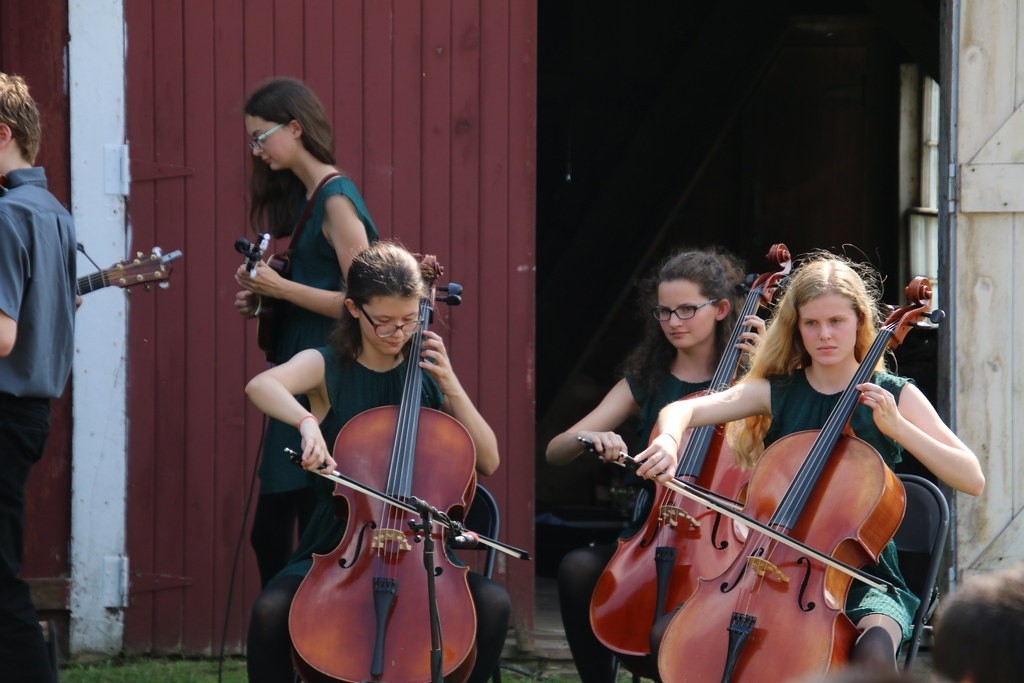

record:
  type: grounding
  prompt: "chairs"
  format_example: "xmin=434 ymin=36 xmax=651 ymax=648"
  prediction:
xmin=893 ymin=472 xmax=949 ymax=681
xmin=291 ymin=484 xmax=499 ymax=683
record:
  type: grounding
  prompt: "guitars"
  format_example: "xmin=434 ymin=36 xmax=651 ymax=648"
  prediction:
xmin=233 ymin=232 xmax=290 ymax=319
xmin=75 ymin=241 xmax=183 ymax=296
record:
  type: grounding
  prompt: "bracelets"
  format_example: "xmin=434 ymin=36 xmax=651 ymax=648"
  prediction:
xmin=662 ymin=430 xmax=680 ymax=450
xmin=297 ymin=414 xmax=319 ymax=431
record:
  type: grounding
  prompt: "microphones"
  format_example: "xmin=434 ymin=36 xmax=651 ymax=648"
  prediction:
xmin=451 ymin=532 xmax=480 ymax=547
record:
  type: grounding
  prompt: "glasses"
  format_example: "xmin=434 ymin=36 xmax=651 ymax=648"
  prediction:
xmin=650 ymin=296 xmax=723 ymax=320
xmin=356 ymin=302 xmax=424 ymax=337
xmin=248 ymin=124 xmax=285 ymax=151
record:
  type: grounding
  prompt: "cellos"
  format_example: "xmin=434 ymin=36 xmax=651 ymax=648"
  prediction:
xmin=588 ymin=243 xmax=946 ymax=683
xmin=288 ymin=254 xmax=478 ymax=683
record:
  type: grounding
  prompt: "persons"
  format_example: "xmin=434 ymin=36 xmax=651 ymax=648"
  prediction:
xmin=0 ymin=72 xmax=82 ymax=683
xmin=235 ymin=76 xmax=379 ymax=591
xmin=928 ymin=565 xmax=1024 ymax=683
xmin=546 ymin=251 xmax=767 ymax=683
xmin=246 ymin=242 xmax=514 ymax=683
xmin=634 ymin=261 xmax=985 ymax=683
